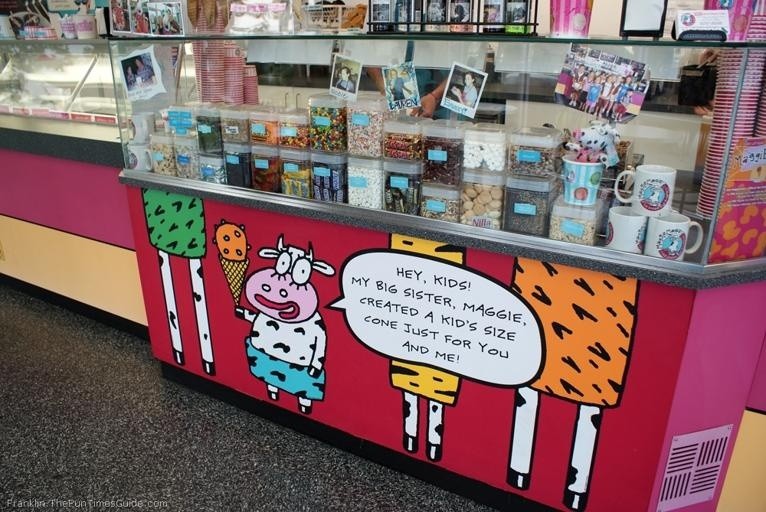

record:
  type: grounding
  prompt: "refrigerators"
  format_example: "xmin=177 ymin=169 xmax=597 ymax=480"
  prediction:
xmin=0 ymin=39 xmax=183 ymax=126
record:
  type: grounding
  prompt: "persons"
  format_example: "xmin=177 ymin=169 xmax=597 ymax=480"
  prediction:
xmin=133 ymin=57 xmax=154 ymax=84
xmin=450 ymin=71 xmax=478 ymax=108
xmin=564 ymin=61 xmax=638 ymax=125
xmin=384 ymin=69 xmax=413 ymax=102
xmin=126 ymin=66 xmax=136 ymax=89
xmin=335 ymin=67 xmax=355 ymax=93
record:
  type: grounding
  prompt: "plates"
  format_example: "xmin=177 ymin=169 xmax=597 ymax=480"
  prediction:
xmin=696 ymin=14 xmax=765 ymax=221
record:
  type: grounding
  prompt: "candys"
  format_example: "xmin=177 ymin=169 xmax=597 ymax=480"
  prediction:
xmin=149 ymin=107 xmax=603 ymax=247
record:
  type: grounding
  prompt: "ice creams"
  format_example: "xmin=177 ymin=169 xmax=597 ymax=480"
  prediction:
xmin=213 ymin=218 xmax=251 ymax=308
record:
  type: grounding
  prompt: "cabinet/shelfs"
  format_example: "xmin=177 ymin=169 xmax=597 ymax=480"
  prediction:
xmin=0 ymin=39 xmax=182 ymax=328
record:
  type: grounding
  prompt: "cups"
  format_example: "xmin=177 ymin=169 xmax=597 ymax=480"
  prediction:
xmin=604 ymin=164 xmax=703 ymax=261
xmin=195 ymin=4 xmax=227 ymax=32
xmin=561 ymin=154 xmax=604 ymax=207
xmin=73 ymin=14 xmax=97 ymax=39
xmin=192 ymin=37 xmax=259 ymax=106
xmin=127 ymin=112 xmax=155 ymax=172
